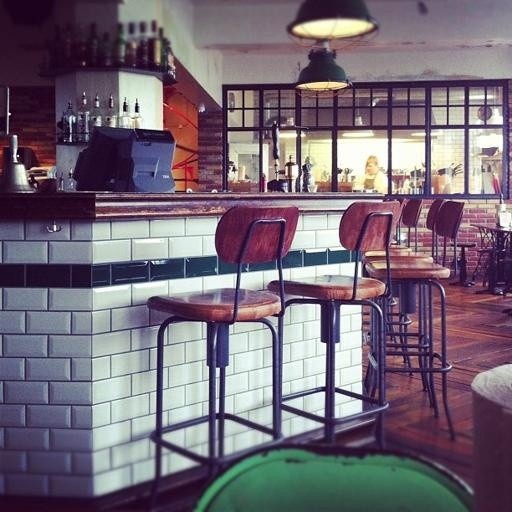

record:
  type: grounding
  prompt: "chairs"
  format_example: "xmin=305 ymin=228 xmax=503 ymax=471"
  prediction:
xmin=194 ymin=443 xmax=479 ymax=512
xmin=143 ymin=205 xmax=299 ymax=511
xmin=269 ymin=202 xmax=401 ymax=449
xmin=364 ymin=201 xmax=466 ymax=440
xmin=363 ymin=199 xmax=447 ymax=407
xmin=372 ymin=196 xmax=422 ymax=377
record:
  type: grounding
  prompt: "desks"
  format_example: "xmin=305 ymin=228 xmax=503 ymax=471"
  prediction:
xmin=468 ymin=222 xmax=512 ymax=296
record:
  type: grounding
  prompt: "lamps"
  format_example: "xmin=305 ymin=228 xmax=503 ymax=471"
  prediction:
xmin=293 ymin=42 xmax=350 ymax=97
xmin=287 ymin=0 xmax=379 ymax=52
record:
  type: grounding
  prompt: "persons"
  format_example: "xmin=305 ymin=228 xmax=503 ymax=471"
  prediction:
xmin=351 ymin=156 xmax=394 ymax=193
xmin=301 ymin=156 xmax=315 ymax=191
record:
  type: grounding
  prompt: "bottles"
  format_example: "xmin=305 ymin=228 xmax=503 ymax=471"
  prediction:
xmin=167 ymin=39 xmax=174 ymax=74
xmin=66 ymin=168 xmax=76 ymax=191
xmin=59 ymin=171 xmax=66 ymax=191
xmin=118 ymin=97 xmax=133 ymax=129
xmin=77 ymin=92 xmax=90 ymax=142
xmin=85 ymin=22 xmax=100 ymax=66
xmin=114 ymin=23 xmax=126 ymax=67
xmin=133 ymin=98 xmax=143 ymax=129
xmin=91 ymin=94 xmax=104 ymax=127
xmin=106 ymin=95 xmax=118 ymax=128
xmin=150 ymin=20 xmax=161 ymax=69
xmin=126 ymin=22 xmax=138 ymax=66
xmin=62 ymin=101 xmax=77 ymax=145
xmin=100 ymin=30 xmax=114 ymax=67
xmin=138 ymin=22 xmax=149 ymax=68
xmin=159 ymin=27 xmax=167 ymax=69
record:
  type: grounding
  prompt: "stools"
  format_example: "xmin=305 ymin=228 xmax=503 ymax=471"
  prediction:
xmin=449 ymin=242 xmax=476 ymax=287
